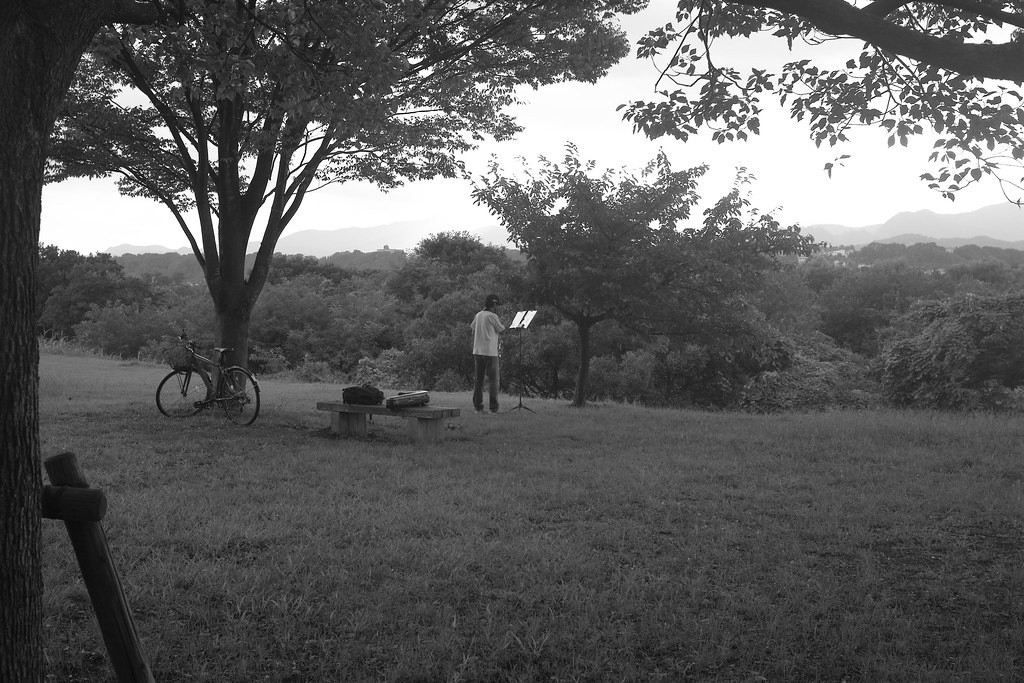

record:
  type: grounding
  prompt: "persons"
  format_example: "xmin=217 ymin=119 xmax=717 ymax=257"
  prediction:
xmin=470 ymin=293 xmax=505 ymax=415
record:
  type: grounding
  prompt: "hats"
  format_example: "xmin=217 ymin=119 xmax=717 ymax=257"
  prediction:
xmin=485 ymin=294 xmax=503 ymax=305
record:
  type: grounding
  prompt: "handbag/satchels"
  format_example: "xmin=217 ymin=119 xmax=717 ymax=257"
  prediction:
xmin=386 ymin=391 xmax=430 ymax=408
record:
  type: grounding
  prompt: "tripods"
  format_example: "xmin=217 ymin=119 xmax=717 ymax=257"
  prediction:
xmin=508 ymin=309 xmax=537 ymax=414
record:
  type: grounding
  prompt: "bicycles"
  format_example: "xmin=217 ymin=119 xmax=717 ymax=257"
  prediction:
xmin=155 ymin=329 xmax=261 ymax=427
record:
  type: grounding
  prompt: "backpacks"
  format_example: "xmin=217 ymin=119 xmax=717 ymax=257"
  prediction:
xmin=342 ymin=383 xmax=385 ymax=406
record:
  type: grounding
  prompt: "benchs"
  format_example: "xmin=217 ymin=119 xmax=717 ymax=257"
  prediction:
xmin=317 ymin=400 xmax=461 ymax=444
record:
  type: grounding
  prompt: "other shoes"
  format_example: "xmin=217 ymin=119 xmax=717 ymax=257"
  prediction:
xmin=489 ymin=410 xmax=502 ymax=414
xmin=475 ymin=409 xmax=488 ymax=414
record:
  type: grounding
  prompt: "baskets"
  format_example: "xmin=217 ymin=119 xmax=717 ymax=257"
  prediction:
xmin=162 ymin=345 xmax=188 ymax=370
